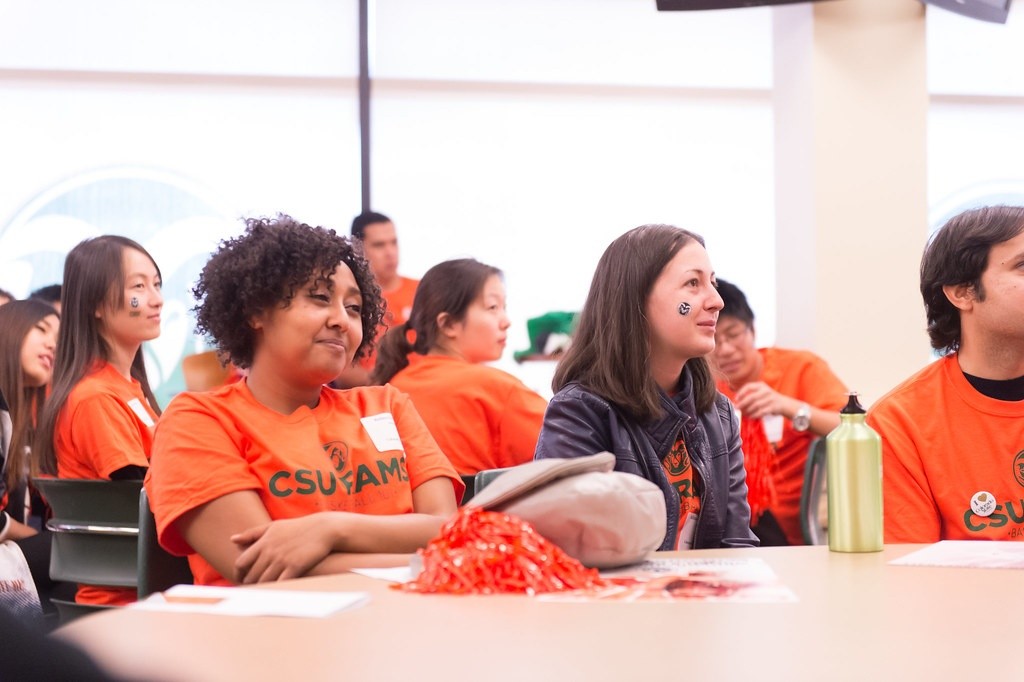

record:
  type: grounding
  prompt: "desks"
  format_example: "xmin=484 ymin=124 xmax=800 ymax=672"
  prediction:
xmin=0 ymin=541 xmax=1024 ymax=682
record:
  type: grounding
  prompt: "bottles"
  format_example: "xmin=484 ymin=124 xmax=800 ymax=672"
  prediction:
xmin=825 ymin=393 xmax=884 ymax=552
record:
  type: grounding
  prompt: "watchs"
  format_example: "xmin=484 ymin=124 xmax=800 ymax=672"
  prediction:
xmin=792 ymin=403 xmax=810 ymax=431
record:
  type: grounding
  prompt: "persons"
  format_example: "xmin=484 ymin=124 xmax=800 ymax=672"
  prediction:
xmin=709 ymin=279 xmax=855 ymax=545
xmin=369 ymin=259 xmax=550 ymax=476
xmin=326 ymin=212 xmax=423 ymax=390
xmin=0 ymin=234 xmax=162 ymax=606
xmin=144 ymin=209 xmax=466 ymax=585
xmin=863 ymin=206 xmax=1024 ymax=546
xmin=532 ymin=224 xmax=759 ymax=550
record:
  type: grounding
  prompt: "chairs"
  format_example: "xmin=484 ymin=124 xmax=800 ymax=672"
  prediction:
xmin=135 ymin=487 xmax=193 ymax=604
xmin=33 ymin=478 xmax=142 ymax=623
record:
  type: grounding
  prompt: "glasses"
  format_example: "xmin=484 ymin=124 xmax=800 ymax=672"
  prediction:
xmin=707 ymin=325 xmax=748 ymax=359
